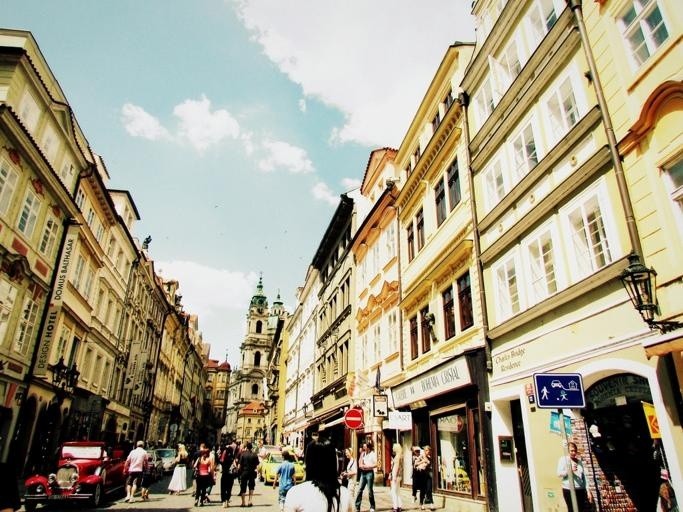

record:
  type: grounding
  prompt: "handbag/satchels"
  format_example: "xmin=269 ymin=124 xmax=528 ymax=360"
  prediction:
xmin=341 ymin=478 xmax=348 ymax=486
xmin=192 ymin=471 xmax=198 ymax=479
xmin=416 ymin=462 xmax=427 ymax=470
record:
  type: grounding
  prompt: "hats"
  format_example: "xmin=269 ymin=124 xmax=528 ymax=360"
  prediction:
xmin=136 ymin=440 xmax=144 ymax=446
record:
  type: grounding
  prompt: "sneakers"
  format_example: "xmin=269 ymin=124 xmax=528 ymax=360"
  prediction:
xmin=240 ymin=503 xmax=253 ymax=506
xmin=124 ymin=495 xmax=149 ymax=503
xmin=194 ymin=498 xmax=210 ymax=506
xmin=169 ymin=491 xmax=179 ymax=495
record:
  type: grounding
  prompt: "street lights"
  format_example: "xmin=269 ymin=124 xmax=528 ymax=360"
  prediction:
xmin=36 ymin=357 xmax=80 ymax=475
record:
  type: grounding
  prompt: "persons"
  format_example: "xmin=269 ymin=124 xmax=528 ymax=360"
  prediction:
xmin=141 ymin=457 xmax=156 ymax=500
xmin=123 ymin=440 xmax=149 ymax=502
xmin=186 ymin=439 xmax=266 ymax=509
xmin=339 ymin=441 xmax=436 ymax=512
xmin=657 ymin=482 xmax=678 ymax=512
xmin=285 ymin=437 xmax=357 ymax=511
xmin=558 ymin=443 xmax=594 ymax=511
xmin=168 ymin=444 xmax=189 ymax=495
xmin=298 ymin=447 xmax=305 ymax=465
xmin=272 ymin=450 xmax=296 ymax=512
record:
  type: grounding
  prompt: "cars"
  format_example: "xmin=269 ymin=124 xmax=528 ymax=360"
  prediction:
xmin=258 ymin=445 xmax=304 ymax=486
xmin=23 ymin=441 xmax=127 ymax=509
xmin=144 ymin=447 xmax=178 ymax=482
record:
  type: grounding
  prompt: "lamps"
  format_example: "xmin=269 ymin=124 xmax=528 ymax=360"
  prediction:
xmin=66 ymin=362 xmax=80 ymax=391
xmin=620 ymin=251 xmax=683 ymax=329
xmin=49 ymin=357 xmax=69 ymax=386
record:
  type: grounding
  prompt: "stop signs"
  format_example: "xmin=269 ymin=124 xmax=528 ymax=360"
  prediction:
xmin=344 ymin=408 xmax=363 ymax=429
xmin=449 ymin=415 xmax=464 ymax=433
